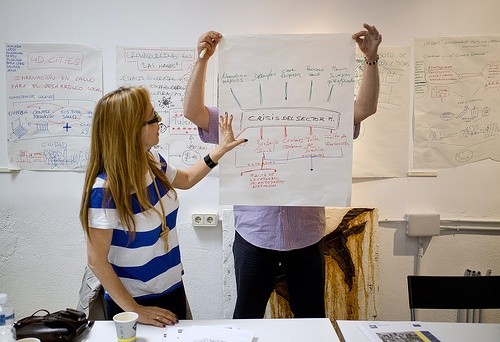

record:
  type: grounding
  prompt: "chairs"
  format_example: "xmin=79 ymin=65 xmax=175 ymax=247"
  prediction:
xmin=406 ymin=272 xmax=500 ymax=325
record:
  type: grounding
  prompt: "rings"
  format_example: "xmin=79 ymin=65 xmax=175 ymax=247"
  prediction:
xmin=157 ymin=315 xmax=160 ymax=320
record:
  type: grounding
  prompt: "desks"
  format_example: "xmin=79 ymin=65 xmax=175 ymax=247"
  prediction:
xmin=0 ymin=312 xmax=500 ymax=342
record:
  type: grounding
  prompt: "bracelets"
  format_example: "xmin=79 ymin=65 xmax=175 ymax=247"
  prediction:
xmin=364 ymin=55 xmax=379 ymax=65
xmin=204 ymin=154 xmax=218 ymax=168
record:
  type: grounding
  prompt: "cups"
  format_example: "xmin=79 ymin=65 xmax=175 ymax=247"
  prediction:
xmin=112 ymin=311 xmax=139 ymax=342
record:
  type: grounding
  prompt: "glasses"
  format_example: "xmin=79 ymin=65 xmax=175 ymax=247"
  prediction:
xmin=140 ymin=111 xmax=161 ymax=128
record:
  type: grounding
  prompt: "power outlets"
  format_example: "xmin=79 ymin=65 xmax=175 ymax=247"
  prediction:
xmin=192 ymin=212 xmax=220 ymax=228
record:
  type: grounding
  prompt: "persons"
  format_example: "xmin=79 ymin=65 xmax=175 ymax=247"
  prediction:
xmin=78 ymin=84 xmax=248 ymax=328
xmin=182 ymin=23 xmax=382 ymax=320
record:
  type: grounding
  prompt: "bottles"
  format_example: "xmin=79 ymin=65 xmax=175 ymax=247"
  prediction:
xmin=0 ymin=293 xmax=17 ymax=342
